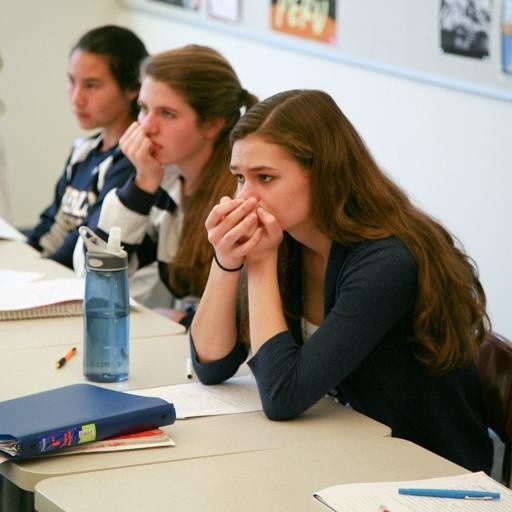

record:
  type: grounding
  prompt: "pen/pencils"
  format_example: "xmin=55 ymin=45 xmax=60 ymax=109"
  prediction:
xmin=55 ymin=346 xmax=76 ymax=368
xmin=398 ymin=488 xmax=501 ymax=501
xmin=185 ymin=357 xmax=192 ymax=379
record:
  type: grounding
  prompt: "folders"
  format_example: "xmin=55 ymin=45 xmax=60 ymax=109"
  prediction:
xmin=0 ymin=382 xmax=176 ymax=461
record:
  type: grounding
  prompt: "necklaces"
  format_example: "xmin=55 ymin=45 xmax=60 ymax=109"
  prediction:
xmin=299 ymin=255 xmax=309 ymax=344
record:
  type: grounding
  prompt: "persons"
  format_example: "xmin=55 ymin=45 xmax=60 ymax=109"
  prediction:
xmin=71 ymin=43 xmax=261 ymax=330
xmin=187 ymin=89 xmax=496 ymax=478
xmin=26 ymin=25 xmax=182 ymax=310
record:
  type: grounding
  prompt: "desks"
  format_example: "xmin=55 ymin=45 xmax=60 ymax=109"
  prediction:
xmin=128 ymin=291 xmax=150 ymax=315
xmin=0 ymin=333 xmax=256 ymax=404
xmin=0 ymin=238 xmax=76 ymax=276
xmin=0 ymin=375 xmax=394 ymax=494
xmin=34 ymin=437 xmax=512 ymax=512
xmin=0 ymin=309 xmax=187 ymax=349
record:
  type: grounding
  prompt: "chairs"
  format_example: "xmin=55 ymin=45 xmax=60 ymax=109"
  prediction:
xmin=474 ymin=327 xmax=511 ymax=489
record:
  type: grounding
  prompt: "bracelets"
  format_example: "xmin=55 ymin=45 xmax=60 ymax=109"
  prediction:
xmin=212 ymin=253 xmax=244 ymax=272
